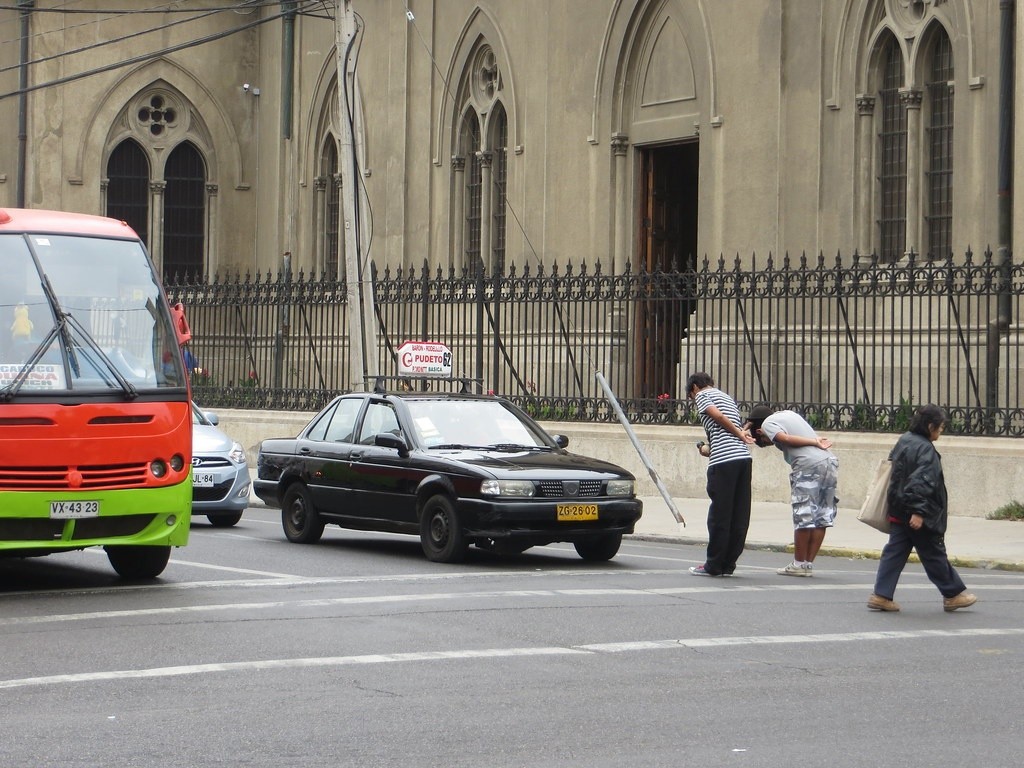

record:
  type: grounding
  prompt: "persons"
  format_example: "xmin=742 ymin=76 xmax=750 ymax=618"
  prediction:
xmin=867 ymin=403 xmax=978 ymax=613
xmin=686 ymin=372 xmax=756 ymax=578
xmin=747 ymin=405 xmax=839 ymax=578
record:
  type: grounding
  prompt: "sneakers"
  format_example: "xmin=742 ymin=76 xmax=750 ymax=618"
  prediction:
xmin=804 ymin=560 xmax=813 ymax=577
xmin=776 ymin=560 xmax=805 ymax=576
xmin=723 ymin=566 xmax=734 ymax=576
xmin=689 ymin=565 xmax=722 ymax=577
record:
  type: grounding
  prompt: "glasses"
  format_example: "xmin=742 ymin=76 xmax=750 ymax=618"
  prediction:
xmin=940 ymin=424 xmax=945 ymax=430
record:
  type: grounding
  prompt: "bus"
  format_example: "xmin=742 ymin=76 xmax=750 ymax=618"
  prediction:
xmin=0 ymin=208 xmax=194 ymax=583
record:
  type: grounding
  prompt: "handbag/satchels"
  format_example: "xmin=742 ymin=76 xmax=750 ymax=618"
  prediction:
xmin=856 ymin=458 xmax=892 ymax=534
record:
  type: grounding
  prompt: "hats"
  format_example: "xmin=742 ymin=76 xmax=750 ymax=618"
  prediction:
xmin=747 ymin=406 xmax=773 ymax=419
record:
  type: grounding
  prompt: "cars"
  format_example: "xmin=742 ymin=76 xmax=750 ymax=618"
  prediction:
xmin=253 ymin=344 xmax=643 ymax=565
xmin=191 ymin=398 xmax=251 ymax=528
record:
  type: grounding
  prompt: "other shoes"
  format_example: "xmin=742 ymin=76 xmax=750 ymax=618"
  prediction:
xmin=867 ymin=592 xmax=900 ymax=611
xmin=943 ymin=592 xmax=978 ymax=611
